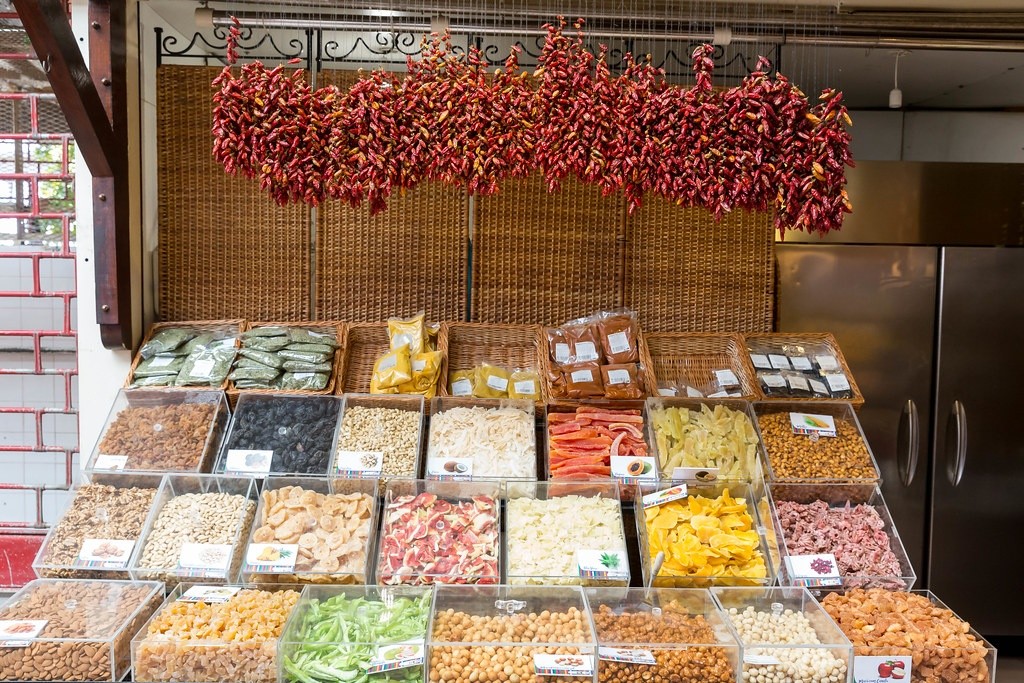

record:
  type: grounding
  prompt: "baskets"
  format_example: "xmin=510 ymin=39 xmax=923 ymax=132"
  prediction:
xmin=124 ymin=319 xmax=864 ymax=419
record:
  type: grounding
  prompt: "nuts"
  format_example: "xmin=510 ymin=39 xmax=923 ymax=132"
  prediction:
xmin=40 ymin=483 xmax=169 ymax=580
xmin=338 ymin=404 xmax=419 ymax=497
xmin=0 ymin=580 xmax=164 ymax=680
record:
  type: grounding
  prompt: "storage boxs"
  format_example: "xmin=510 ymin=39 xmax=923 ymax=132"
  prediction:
xmin=424 ymin=398 xmax=537 ymax=503
xmin=330 ymin=393 xmax=427 ymax=497
xmin=373 ymin=480 xmax=502 ymax=604
xmin=83 ymin=388 xmax=231 ymax=495
xmin=130 ymin=473 xmax=261 ymax=595
xmin=542 ymin=327 xmax=865 ymax=418
xmin=632 ymin=481 xmax=775 ymax=614
xmin=641 ymin=399 xmax=773 ymax=500
xmin=544 ymin=399 xmax=659 ymax=505
xmin=123 ymin=317 xmax=247 ymax=407
xmin=764 ymin=482 xmax=917 ymax=598
xmin=215 ymin=393 xmax=345 ymax=492
xmin=0 ymin=579 xmax=998 ymax=683
xmin=438 ymin=321 xmax=543 ymax=421
xmin=336 ymin=321 xmax=443 ymax=409
xmin=748 ymin=399 xmax=882 ymax=501
xmin=32 ymin=470 xmax=173 ymax=581
xmin=238 ymin=476 xmax=380 ymax=593
xmin=505 ymin=480 xmax=631 ymax=599
xmin=227 ymin=320 xmax=344 ymax=413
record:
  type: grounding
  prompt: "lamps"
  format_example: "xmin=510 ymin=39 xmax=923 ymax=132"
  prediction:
xmin=376 ymin=47 xmax=396 ymax=95
xmin=887 ymin=50 xmax=913 ymax=108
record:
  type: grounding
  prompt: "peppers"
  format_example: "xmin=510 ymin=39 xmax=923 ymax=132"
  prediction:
xmin=210 ymin=15 xmax=854 ymax=242
